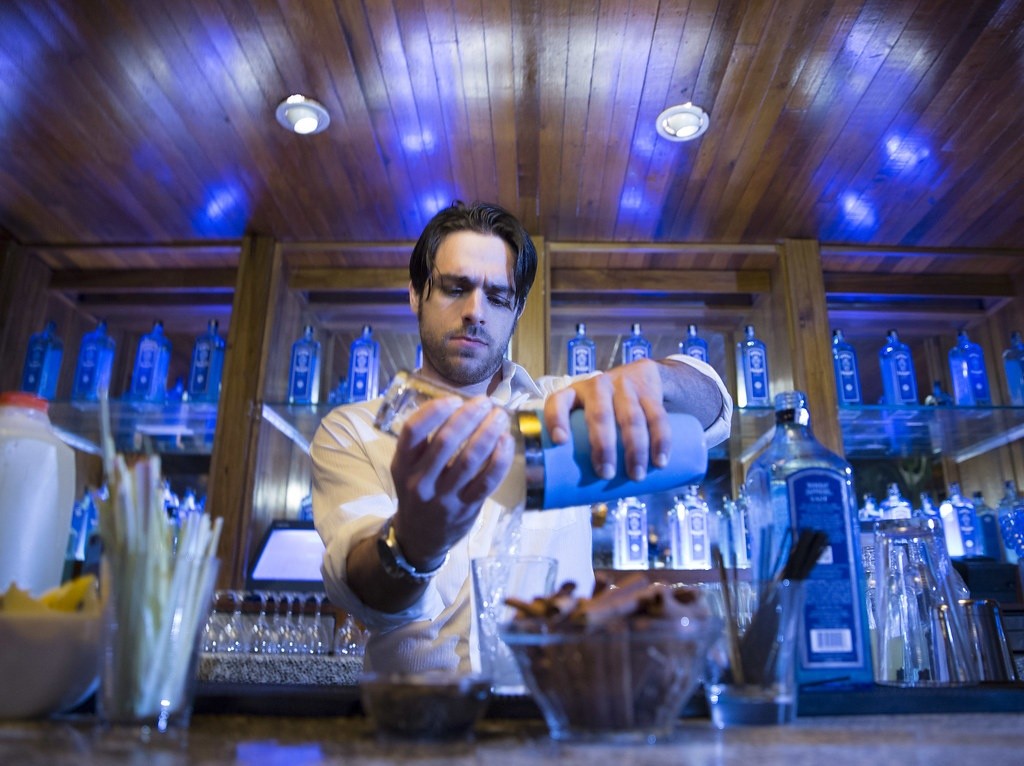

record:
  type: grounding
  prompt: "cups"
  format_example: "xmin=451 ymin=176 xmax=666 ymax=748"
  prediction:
xmin=94 ymin=547 xmax=220 ymax=749
xmin=371 ymin=373 xmax=528 ymax=513
xmin=470 ymin=556 xmax=557 ymax=696
xmin=519 ymin=403 xmax=706 ymax=512
xmin=694 ymin=580 xmax=805 ymax=725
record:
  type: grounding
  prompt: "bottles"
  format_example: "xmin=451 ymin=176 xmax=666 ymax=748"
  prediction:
xmin=1001 ymin=330 xmax=1024 ymax=406
xmin=566 ymin=322 xmax=596 ymax=376
xmin=859 ymin=480 xmax=1024 ymax=576
xmin=678 ymin=324 xmax=708 ymax=366
xmin=948 ymin=329 xmax=992 ymax=419
xmin=0 ymin=390 xmax=77 ymax=604
xmin=747 ymin=394 xmax=872 ymax=692
xmin=738 ymin=324 xmax=771 ymax=416
xmin=833 ymin=329 xmax=862 ymax=421
xmin=672 ymin=483 xmax=756 ymax=569
xmin=621 ymin=324 xmax=651 ymax=365
xmin=613 ymin=494 xmax=650 ymax=570
xmin=878 ymin=329 xmax=920 ymax=434
xmin=931 ymin=380 xmax=958 ymax=437
xmin=0 ymin=313 xmax=424 ymax=557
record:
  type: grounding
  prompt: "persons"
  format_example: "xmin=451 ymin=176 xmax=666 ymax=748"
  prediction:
xmin=310 ymin=197 xmax=733 ymax=700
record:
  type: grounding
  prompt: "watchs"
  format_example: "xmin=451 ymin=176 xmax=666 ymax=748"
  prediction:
xmin=374 ymin=514 xmax=449 ymax=588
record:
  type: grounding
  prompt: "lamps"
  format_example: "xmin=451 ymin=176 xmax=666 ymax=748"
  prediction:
xmin=275 ymin=94 xmax=331 ymax=135
xmin=655 ymin=104 xmax=709 ymax=142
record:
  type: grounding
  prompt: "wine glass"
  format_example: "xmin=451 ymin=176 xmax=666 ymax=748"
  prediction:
xmin=198 ymin=585 xmax=375 ymax=658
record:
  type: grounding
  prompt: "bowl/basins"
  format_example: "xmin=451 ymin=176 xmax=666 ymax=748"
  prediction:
xmin=497 ymin=618 xmax=719 ymax=744
xmin=0 ymin=609 xmax=106 ymax=719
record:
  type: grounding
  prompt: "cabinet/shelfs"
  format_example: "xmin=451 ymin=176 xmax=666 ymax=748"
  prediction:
xmin=0 ymin=237 xmax=1024 ymax=713
